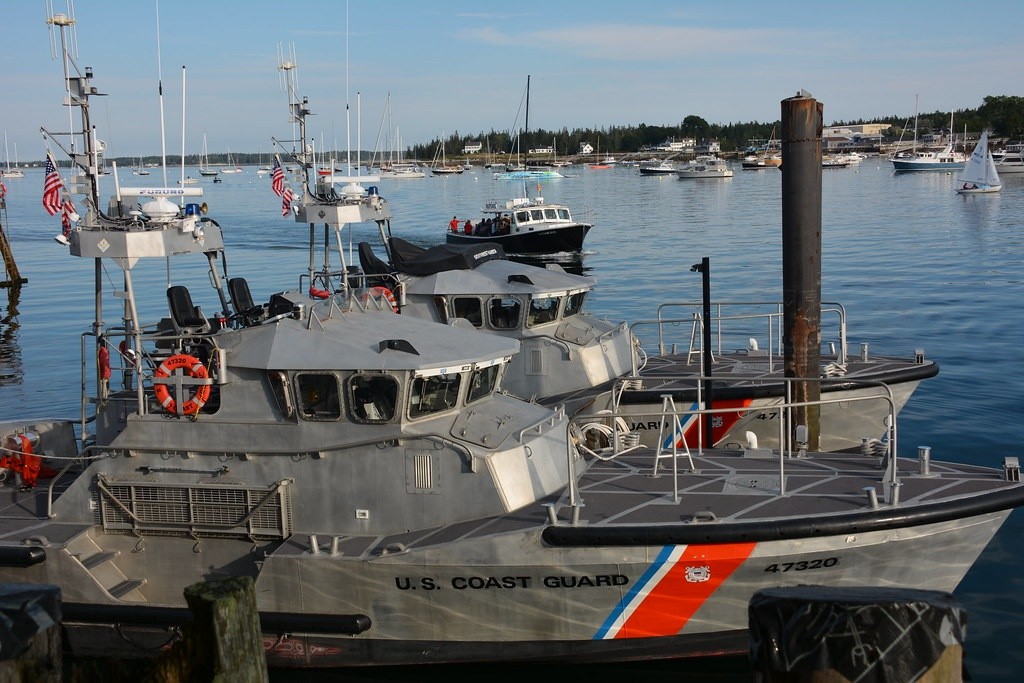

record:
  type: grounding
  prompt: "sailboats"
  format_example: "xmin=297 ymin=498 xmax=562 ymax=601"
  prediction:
xmin=889 ymin=92 xmax=969 ymax=171
xmin=954 ymin=129 xmax=1003 ymax=196
xmin=0 ymin=126 xmax=25 ymax=179
xmin=484 ymin=76 xmax=568 ymax=181
xmin=545 ymin=137 xmax=572 ymax=167
xmin=764 ymin=126 xmax=782 ymax=167
xmin=307 ymin=0 xmax=427 ymax=184
xmin=0 ymin=0 xmax=1024 ymax=683
xmin=100 ymin=66 xmax=295 ymax=175
xmin=431 ymin=132 xmax=473 ymax=174
xmin=445 ymin=196 xmax=594 ymax=252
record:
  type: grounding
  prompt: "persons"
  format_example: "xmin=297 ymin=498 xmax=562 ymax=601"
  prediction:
xmin=448 ymin=214 xmax=492 ymax=237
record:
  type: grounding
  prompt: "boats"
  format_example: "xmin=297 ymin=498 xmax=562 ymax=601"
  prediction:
xmin=177 ymin=179 xmax=197 ymax=184
xmin=676 ymin=155 xmax=735 ymax=178
xmin=992 ymin=141 xmax=1024 ymax=174
xmin=214 ymin=179 xmax=221 ymax=184
xmin=822 ymin=152 xmax=880 ymax=166
xmin=129 ymin=40 xmax=943 ymax=456
xmin=741 ymin=156 xmax=764 ymax=167
xmin=639 ymin=158 xmax=678 ymax=173
xmin=592 ymin=150 xmax=640 ymax=168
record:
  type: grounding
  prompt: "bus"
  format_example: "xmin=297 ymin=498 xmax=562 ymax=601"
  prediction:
xmin=851 ymin=134 xmax=885 ymax=144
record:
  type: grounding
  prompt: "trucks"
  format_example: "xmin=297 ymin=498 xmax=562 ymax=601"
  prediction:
xmin=747 ymin=148 xmax=766 ymax=153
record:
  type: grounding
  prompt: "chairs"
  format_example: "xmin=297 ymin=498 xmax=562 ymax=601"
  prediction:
xmin=357 ymin=242 xmax=395 ymax=283
xmin=167 ymin=285 xmax=206 ymax=327
xmin=388 ymin=237 xmax=427 ymax=272
xmin=228 ymin=277 xmax=266 ymax=320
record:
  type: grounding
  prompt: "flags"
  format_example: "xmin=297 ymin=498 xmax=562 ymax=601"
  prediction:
xmin=272 ymin=156 xmax=286 ymax=196
xmin=280 ymin=181 xmax=295 ymax=219
xmin=61 ymin=190 xmax=79 ymax=241
xmin=42 ymin=149 xmax=63 ymax=215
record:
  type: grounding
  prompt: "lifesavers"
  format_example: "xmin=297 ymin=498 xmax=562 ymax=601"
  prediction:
xmin=0 ymin=183 xmax=7 ymax=198
xmin=538 ymin=184 xmax=541 ymax=191
xmin=364 ymin=287 xmax=397 ymax=313
xmin=155 ymin=355 xmax=211 ymax=414
